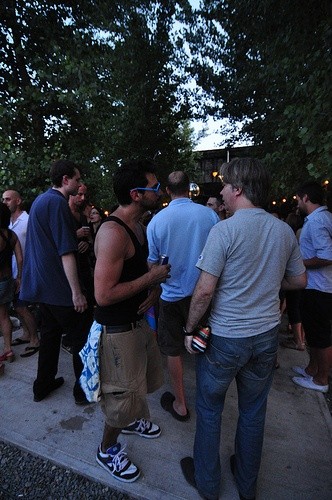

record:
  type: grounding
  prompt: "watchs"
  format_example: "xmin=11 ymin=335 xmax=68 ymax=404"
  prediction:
xmin=183 ymin=326 xmax=195 ymax=336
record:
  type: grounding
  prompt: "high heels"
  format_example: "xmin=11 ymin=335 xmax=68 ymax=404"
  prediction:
xmin=0 ymin=349 xmax=15 ymax=362
xmin=0 ymin=361 xmax=6 ymax=376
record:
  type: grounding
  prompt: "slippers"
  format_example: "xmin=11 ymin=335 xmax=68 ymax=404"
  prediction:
xmin=160 ymin=390 xmax=191 ymax=422
xmin=20 ymin=346 xmax=40 ymax=357
xmin=11 ymin=338 xmax=30 ymax=346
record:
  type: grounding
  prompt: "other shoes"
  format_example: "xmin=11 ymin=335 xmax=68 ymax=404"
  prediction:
xmin=33 ymin=376 xmax=64 ymax=402
xmin=72 ymin=380 xmax=90 ymax=404
xmin=180 ymin=457 xmax=201 ymax=490
xmin=62 ymin=345 xmax=73 ymax=356
xmin=230 ymin=454 xmax=256 ymax=500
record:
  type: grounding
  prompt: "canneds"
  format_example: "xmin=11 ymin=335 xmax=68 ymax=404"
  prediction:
xmin=158 ymin=255 xmax=168 ymax=265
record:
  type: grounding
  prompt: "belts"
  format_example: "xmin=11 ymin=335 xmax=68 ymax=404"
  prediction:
xmin=102 ymin=321 xmax=136 ymax=334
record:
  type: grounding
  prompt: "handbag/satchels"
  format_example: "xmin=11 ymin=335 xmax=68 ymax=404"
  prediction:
xmin=190 ymin=323 xmax=212 ymax=354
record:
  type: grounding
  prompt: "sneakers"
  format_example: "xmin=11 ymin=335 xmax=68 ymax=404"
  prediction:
xmin=96 ymin=441 xmax=141 ymax=483
xmin=291 ymin=365 xmax=314 ymax=378
xmin=120 ymin=416 xmax=162 ymax=438
xmin=291 ymin=376 xmax=329 ymax=394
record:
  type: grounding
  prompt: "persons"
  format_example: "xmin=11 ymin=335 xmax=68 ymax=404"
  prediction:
xmin=0 ymin=190 xmax=40 ymax=376
xmin=146 ymin=169 xmax=221 ymax=422
xmin=92 ymin=160 xmax=163 ymax=483
xmin=204 ymin=194 xmax=226 ymax=221
xmin=291 ymin=177 xmax=332 ymax=393
xmin=180 ymin=157 xmax=306 ymax=500
xmin=67 ymin=183 xmax=104 ymax=258
xmin=281 ymin=213 xmax=304 ymax=351
xmin=19 ymin=159 xmax=104 ymax=405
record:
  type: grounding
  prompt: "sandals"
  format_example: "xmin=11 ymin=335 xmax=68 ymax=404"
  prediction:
xmin=279 ymin=336 xmax=306 ymax=351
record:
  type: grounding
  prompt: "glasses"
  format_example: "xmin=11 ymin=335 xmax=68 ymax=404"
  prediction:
xmin=131 ymin=182 xmax=161 ymax=194
xmin=91 ymin=211 xmax=101 ymax=215
